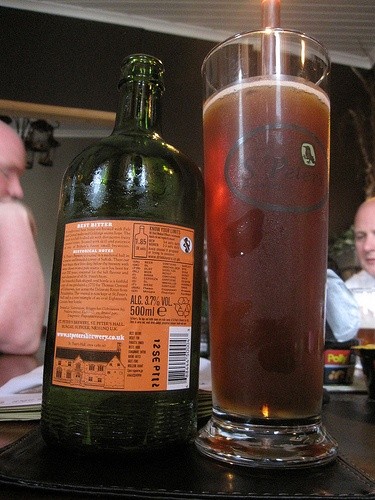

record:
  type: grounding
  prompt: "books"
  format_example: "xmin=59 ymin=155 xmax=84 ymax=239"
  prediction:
xmin=0 ymin=356 xmax=213 ymax=422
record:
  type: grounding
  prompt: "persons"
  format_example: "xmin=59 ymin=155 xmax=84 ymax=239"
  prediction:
xmin=0 ymin=122 xmax=44 ymax=354
xmin=343 ymin=197 xmax=375 ymax=330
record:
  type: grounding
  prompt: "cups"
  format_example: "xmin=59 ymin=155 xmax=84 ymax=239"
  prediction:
xmin=196 ymin=27 xmax=341 ymax=470
xmin=360 ymin=348 xmax=374 ymax=403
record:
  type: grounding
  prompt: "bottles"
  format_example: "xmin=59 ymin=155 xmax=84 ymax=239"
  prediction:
xmin=40 ymin=54 xmax=205 ymax=457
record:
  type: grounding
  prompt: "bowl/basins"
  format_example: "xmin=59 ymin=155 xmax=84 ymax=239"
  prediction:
xmin=324 ymin=338 xmax=359 ymax=385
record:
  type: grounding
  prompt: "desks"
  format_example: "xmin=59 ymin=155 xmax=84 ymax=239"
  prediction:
xmin=0 ymin=346 xmax=374 ymax=500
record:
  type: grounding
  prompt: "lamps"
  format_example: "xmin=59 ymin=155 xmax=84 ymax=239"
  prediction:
xmin=0 ymin=115 xmax=61 ymax=169
